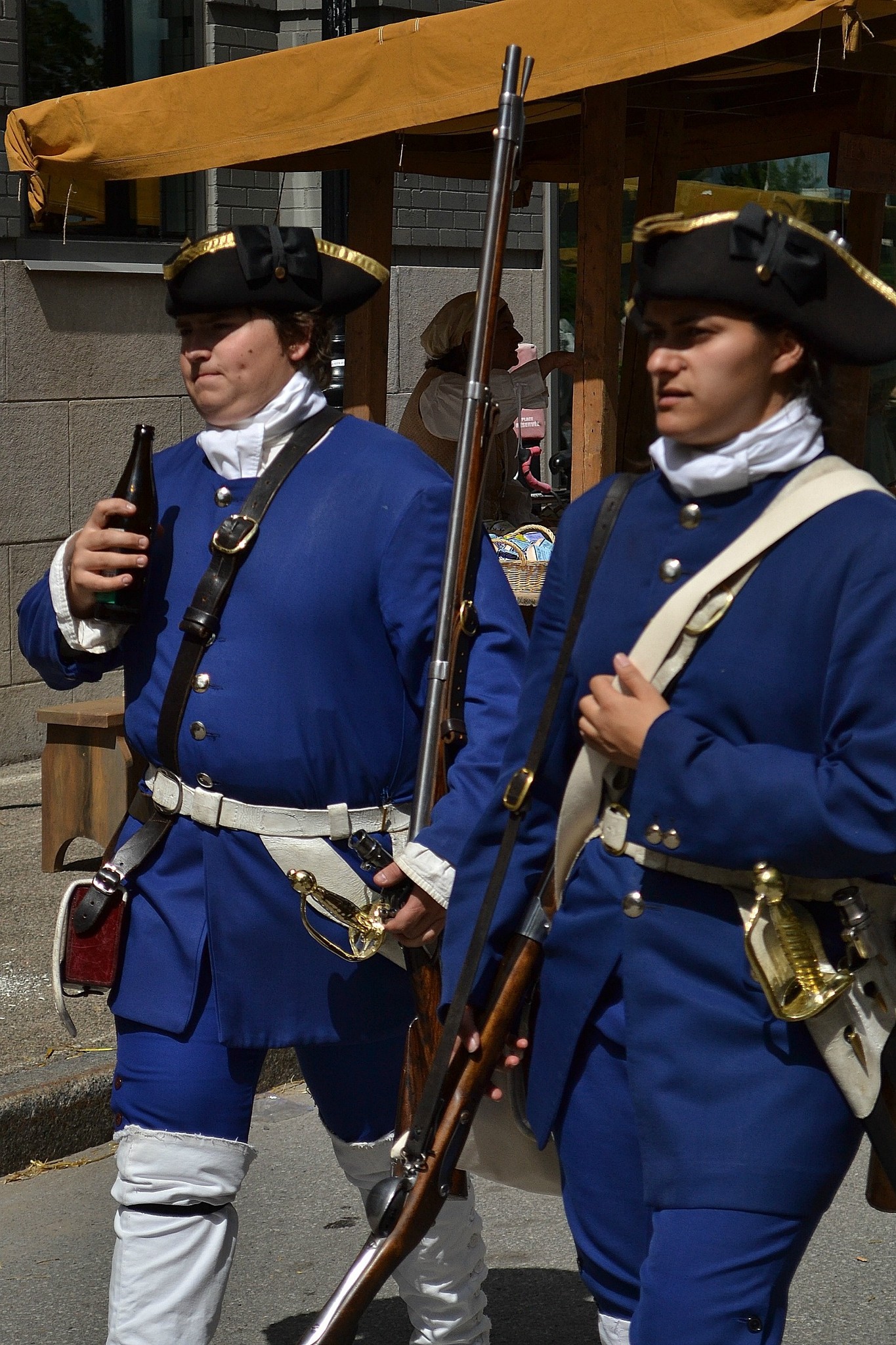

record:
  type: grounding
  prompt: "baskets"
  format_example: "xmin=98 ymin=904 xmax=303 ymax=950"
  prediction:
xmin=491 ymin=524 xmax=556 ymax=592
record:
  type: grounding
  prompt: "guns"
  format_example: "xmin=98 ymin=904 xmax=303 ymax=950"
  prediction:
xmin=393 ymin=43 xmax=537 ymax=1163
xmin=301 ymin=855 xmax=558 ymax=1345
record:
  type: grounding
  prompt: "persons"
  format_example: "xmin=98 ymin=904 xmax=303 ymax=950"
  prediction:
xmin=18 ymin=210 xmax=895 ymax=1345
xmin=18 ymin=223 xmax=537 ymax=1345
xmin=394 ymin=292 xmax=552 ymax=524
xmin=440 ymin=209 xmax=894 ymax=1344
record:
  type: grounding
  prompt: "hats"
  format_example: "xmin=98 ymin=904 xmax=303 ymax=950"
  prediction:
xmin=164 ymin=221 xmax=388 ymax=316
xmin=630 ymin=213 xmax=896 ymax=377
xmin=421 ymin=291 xmax=509 ymax=358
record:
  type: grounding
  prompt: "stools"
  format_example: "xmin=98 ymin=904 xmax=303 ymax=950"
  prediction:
xmin=37 ymin=697 xmax=138 ymax=874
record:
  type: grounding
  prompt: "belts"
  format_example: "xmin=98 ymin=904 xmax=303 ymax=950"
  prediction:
xmin=145 ymin=765 xmax=416 ymax=839
xmin=598 ymin=808 xmax=859 ymax=904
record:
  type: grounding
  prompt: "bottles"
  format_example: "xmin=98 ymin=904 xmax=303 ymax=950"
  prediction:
xmin=96 ymin=424 xmax=159 ymax=624
